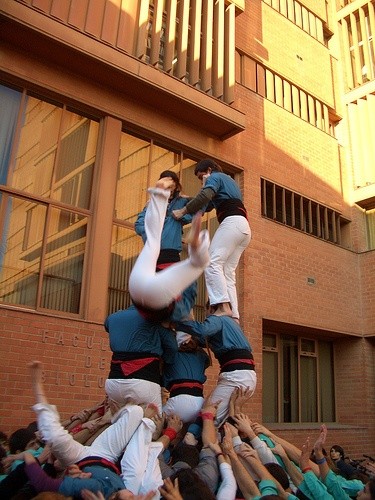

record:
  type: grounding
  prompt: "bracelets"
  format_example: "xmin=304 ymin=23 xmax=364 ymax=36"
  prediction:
xmin=164 ymin=427 xmax=177 ymax=441
xmin=202 ymin=413 xmax=214 ymax=421
xmin=70 ymin=416 xmax=74 ymax=422
xmin=301 ymin=464 xmax=312 ymax=473
xmin=316 ymin=458 xmax=326 ymax=464
xmin=216 ymin=452 xmax=225 ymax=458
xmin=70 ymin=423 xmax=82 ymax=434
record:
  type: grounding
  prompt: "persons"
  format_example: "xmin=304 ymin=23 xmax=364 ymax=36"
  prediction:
xmin=104 ymin=303 xmax=177 ymax=418
xmin=171 ymin=158 xmax=252 ymax=325
xmin=0 ymin=386 xmax=375 ymax=500
xmin=25 ymin=361 xmax=144 ymax=500
xmin=129 ymin=175 xmax=212 ymax=326
xmin=164 ymin=298 xmax=258 ymax=427
xmin=133 ymin=170 xmax=216 ymax=273
xmin=162 ymin=328 xmax=213 ymax=425
